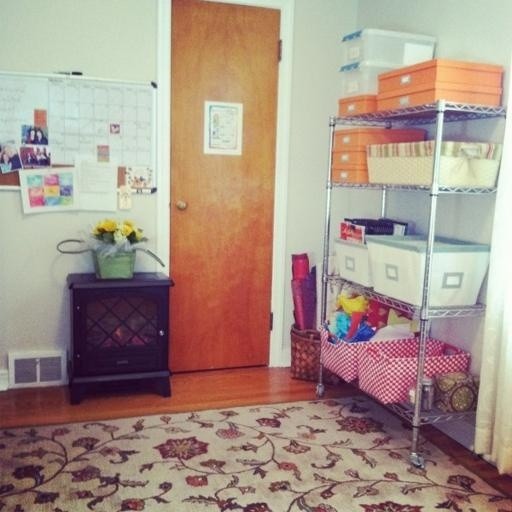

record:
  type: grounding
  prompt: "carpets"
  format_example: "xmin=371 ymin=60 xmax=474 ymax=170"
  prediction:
xmin=0 ymin=395 xmax=512 ymax=512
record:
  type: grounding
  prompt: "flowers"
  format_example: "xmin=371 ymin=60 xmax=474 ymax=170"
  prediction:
xmin=83 ymin=217 xmax=145 ymax=254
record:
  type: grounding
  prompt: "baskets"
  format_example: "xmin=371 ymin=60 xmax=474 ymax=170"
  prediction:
xmin=290 ymin=323 xmax=343 ymax=386
xmin=57 ymin=240 xmax=165 ymax=280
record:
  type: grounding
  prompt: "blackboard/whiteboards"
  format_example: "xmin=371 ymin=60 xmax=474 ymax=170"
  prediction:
xmin=0 ymin=71 xmax=157 ymax=195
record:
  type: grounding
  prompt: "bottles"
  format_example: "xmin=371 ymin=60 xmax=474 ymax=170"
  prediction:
xmin=422 ymin=378 xmax=434 ymax=409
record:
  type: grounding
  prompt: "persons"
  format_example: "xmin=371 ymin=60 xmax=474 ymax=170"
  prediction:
xmin=25 ymin=129 xmax=47 ymax=145
xmin=0 ymin=145 xmax=22 ymax=173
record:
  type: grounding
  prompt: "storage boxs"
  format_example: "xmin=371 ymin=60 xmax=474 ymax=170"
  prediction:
xmin=323 ymin=331 xmax=470 ymax=405
xmin=335 ymin=232 xmax=490 ymax=309
xmin=377 ymin=61 xmax=503 ymax=108
xmin=367 ymin=140 xmax=501 ymax=190
xmin=332 ymin=129 xmax=425 ymax=182
xmin=340 ymin=30 xmax=437 ymax=95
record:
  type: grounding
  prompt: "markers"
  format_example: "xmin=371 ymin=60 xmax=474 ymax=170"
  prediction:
xmin=58 ymin=71 xmax=83 ymax=76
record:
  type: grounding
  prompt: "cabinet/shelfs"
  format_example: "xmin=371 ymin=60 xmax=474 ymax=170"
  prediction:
xmin=326 ymin=100 xmax=508 ymax=470
xmin=339 ymin=95 xmax=377 ymax=115
xmin=65 ymin=270 xmax=176 ymax=405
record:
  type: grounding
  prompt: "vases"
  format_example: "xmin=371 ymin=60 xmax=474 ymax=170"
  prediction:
xmin=91 ymin=250 xmax=137 ymax=280
